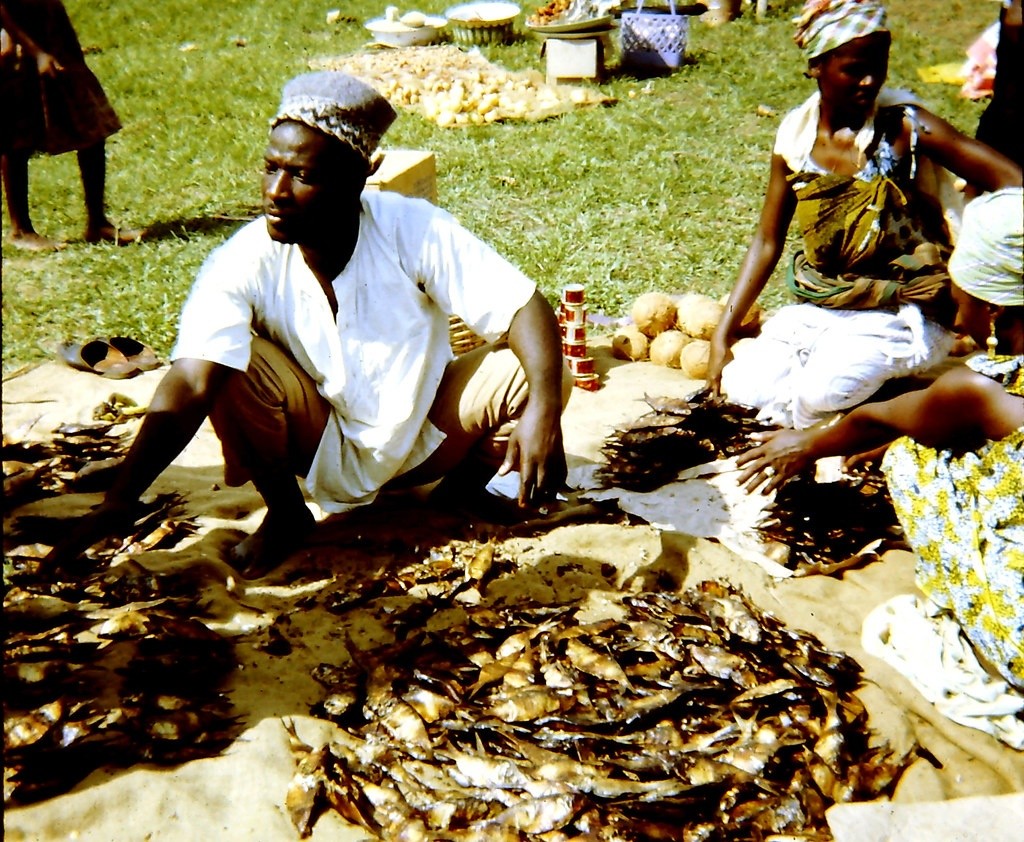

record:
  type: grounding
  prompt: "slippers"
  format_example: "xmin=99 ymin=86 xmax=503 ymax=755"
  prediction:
xmin=85 ymin=335 xmax=161 ymax=372
xmin=65 ymin=339 xmax=139 ymax=379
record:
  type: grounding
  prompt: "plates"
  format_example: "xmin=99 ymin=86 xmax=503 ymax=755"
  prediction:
xmin=525 ymin=14 xmax=612 ymax=33
xmin=532 ymin=30 xmax=619 ymax=39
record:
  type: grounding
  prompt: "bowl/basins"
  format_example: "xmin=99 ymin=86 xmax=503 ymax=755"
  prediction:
xmin=364 ymin=17 xmax=448 ymax=47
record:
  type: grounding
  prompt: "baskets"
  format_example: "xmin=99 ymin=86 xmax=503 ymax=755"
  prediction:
xmin=618 ymin=0 xmax=690 ymax=68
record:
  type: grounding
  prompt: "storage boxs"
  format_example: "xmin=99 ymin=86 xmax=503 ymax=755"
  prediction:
xmin=535 ymin=28 xmax=610 ymax=88
xmin=361 ymin=149 xmax=439 ymax=208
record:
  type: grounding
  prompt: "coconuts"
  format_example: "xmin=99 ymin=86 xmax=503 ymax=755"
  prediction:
xmin=611 ymin=291 xmax=760 ymax=380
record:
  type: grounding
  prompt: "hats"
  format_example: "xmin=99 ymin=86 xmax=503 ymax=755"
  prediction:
xmin=792 ymin=0 xmax=889 ymax=60
xmin=946 ymin=185 xmax=1024 ymax=306
xmin=269 ymin=71 xmax=398 ymax=160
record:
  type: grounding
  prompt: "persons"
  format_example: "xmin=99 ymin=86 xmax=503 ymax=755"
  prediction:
xmin=33 ymin=73 xmax=573 ymax=579
xmin=735 ymin=187 xmax=1024 ymax=750
xmin=946 ymin=0 xmax=1024 ymax=203
xmin=705 ymin=0 xmax=1024 ymax=400
xmin=0 ymin=0 xmax=162 ymax=251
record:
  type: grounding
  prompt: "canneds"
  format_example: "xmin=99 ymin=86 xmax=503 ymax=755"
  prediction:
xmin=553 ymin=283 xmax=599 ymax=392
